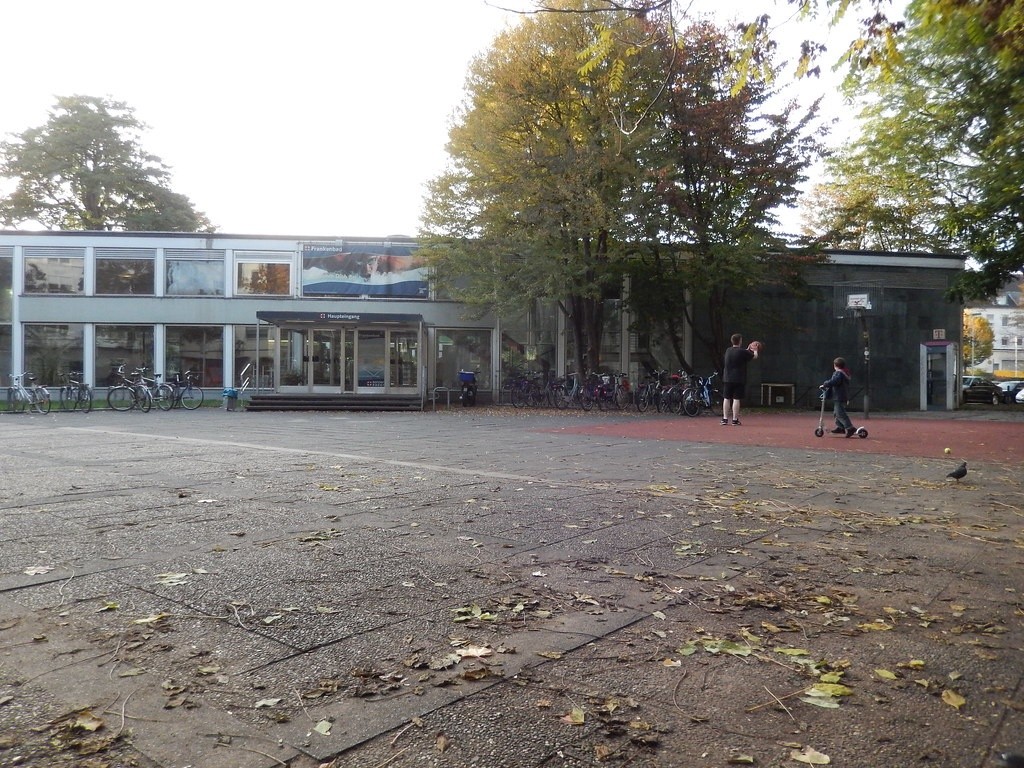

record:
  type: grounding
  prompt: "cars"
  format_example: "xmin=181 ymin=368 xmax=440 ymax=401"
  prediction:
xmin=996 ymin=381 xmax=1024 ymax=403
xmin=961 ymin=376 xmax=1004 ymax=404
xmin=1016 ymin=388 xmax=1024 ymax=404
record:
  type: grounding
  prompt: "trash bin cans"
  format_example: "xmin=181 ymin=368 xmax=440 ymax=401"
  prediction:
xmin=223 ymin=389 xmax=238 ymax=412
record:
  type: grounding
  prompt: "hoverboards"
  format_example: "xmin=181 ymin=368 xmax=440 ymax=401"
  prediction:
xmin=815 ymin=385 xmax=868 ymax=439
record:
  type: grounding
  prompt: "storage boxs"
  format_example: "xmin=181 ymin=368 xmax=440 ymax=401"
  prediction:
xmin=458 ymin=371 xmax=474 ymax=382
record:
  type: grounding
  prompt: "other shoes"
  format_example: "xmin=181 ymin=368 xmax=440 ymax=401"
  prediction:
xmin=832 ymin=427 xmax=845 ymax=434
xmin=846 ymin=427 xmax=857 ymax=438
xmin=732 ymin=420 xmax=741 ymax=425
xmin=721 ymin=420 xmax=728 ymax=425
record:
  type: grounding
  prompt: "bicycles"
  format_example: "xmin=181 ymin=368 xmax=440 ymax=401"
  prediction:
xmin=108 ymin=364 xmax=205 ymax=414
xmin=510 ymin=371 xmax=724 ymax=418
xmin=7 ymin=371 xmax=51 ymax=416
xmin=59 ymin=372 xmax=94 ymax=413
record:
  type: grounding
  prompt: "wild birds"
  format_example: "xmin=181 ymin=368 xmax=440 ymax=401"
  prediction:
xmin=946 ymin=461 xmax=967 ymax=482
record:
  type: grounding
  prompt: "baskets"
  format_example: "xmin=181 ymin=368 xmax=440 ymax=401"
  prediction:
xmin=459 ymin=372 xmax=474 ymax=382
xmin=618 ymin=377 xmax=629 ymax=386
xmin=602 ymin=377 xmax=610 ymax=385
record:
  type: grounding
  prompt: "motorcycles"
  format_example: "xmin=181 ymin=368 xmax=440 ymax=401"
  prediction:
xmin=459 ymin=364 xmax=481 ymax=406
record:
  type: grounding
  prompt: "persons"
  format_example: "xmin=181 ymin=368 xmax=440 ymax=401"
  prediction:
xmin=189 ymin=362 xmax=198 ymax=377
xmin=719 ymin=334 xmax=758 ymax=426
xmin=820 ymin=358 xmax=857 ymax=438
xmin=177 ymin=369 xmax=182 ymax=381
xmin=47 ymin=369 xmax=54 ymax=387
xmin=541 ymin=358 xmax=550 ymax=388
